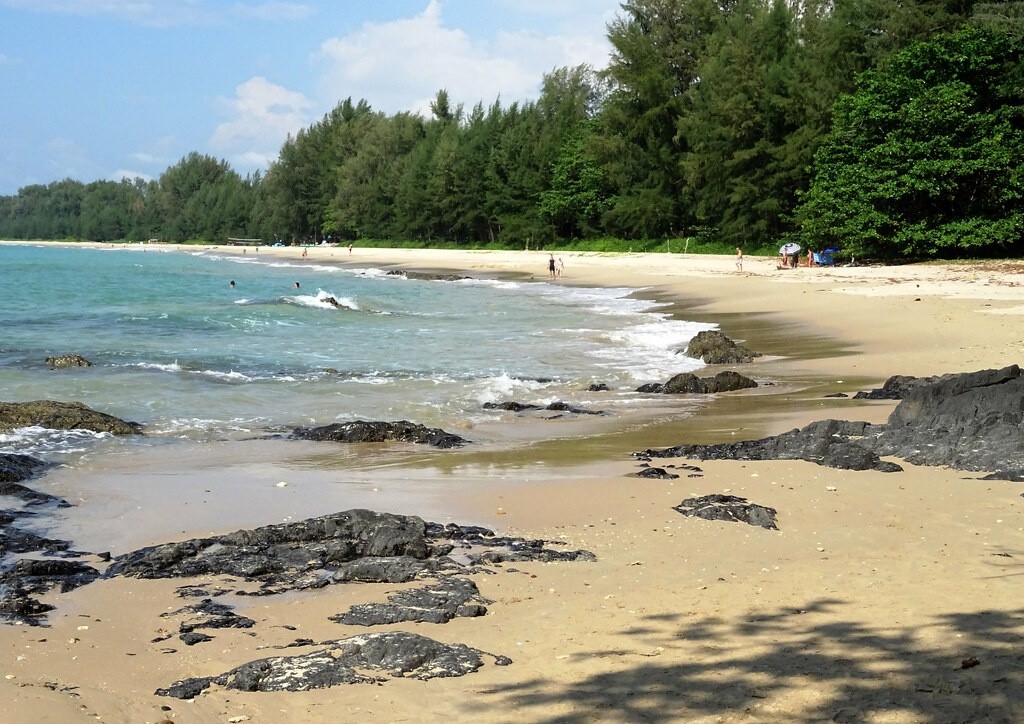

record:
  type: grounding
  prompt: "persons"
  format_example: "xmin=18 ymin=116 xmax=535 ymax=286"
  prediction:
xmin=230 ymin=281 xmax=235 ymax=288
xmin=735 ymin=246 xmax=744 ymax=273
xmin=301 ymin=248 xmax=308 ymax=259
xmin=295 ymin=282 xmax=299 ymax=288
xmin=348 ymin=243 xmax=352 ymax=255
xmin=783 ymin=247 xmax=814 ymax=269
xmin=547 ymin=253 xmax=564 ymax=280
